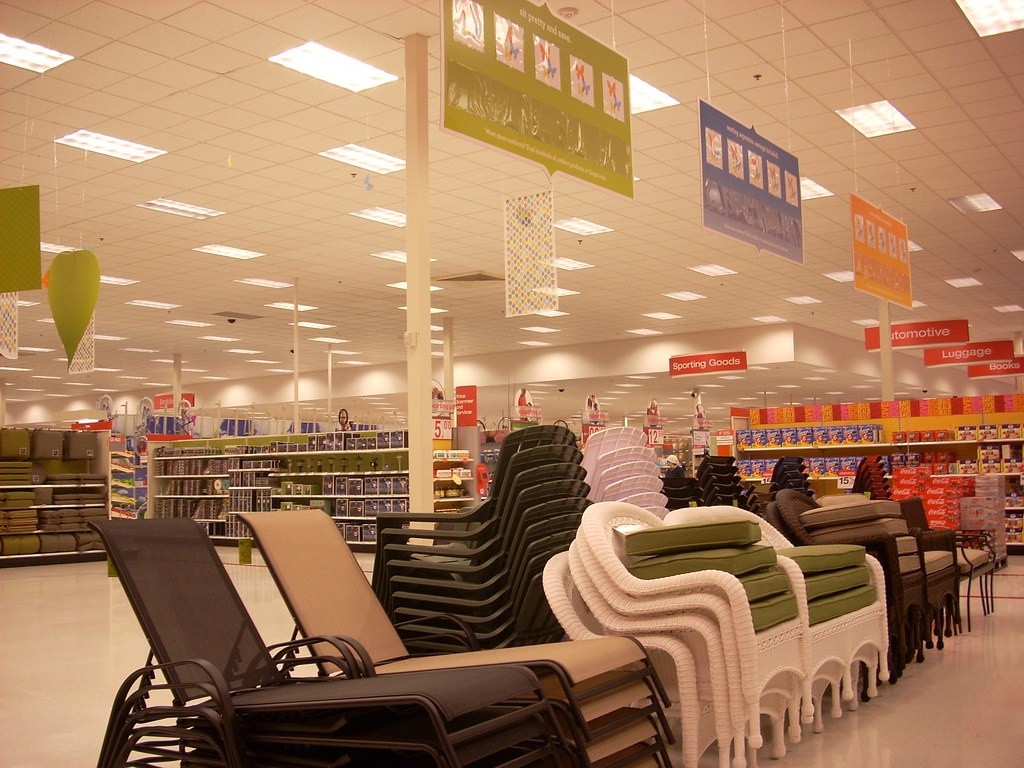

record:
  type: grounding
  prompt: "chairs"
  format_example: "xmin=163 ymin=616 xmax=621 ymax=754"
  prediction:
xmin=96 ymin=425 xmax=996 ymax=768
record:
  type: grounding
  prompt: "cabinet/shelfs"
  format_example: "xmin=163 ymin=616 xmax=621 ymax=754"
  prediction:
xmin=736 ymin=421 xmax=883 ymax=485
xmin=109 ymin=451 xmax=147 ymax=520
xmin=433 ymin=459 xmax=474 ymax=512
xmin=147 ymin=429 xmax=408 ymax=544
xmin=481 ymin=453 xmax=499 ymax=500
xmin=0 ymin=430 xmax=111 ymax=559
xmin=889 ymin=433 xmax=1023 ymax=548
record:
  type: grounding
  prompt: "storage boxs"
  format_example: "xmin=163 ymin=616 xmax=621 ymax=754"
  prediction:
xmin=155 ymin=431 xmax=409 ymax=542
xmin=736 ymin=425 xmax=891 ymax=479
xmin=888 ymin=424 xmax=1024 ymax=562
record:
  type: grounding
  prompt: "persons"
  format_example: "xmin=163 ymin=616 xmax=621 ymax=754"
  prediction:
xmin=647 ymin=400 xmax=659 ymax=426
xmin=519 ymin=388 xmax=532 ymax=422
xmin=695 ymin=404 xmax=707 ymax=429
xmin=588 ymin=393 xmax=598 ymax=425
